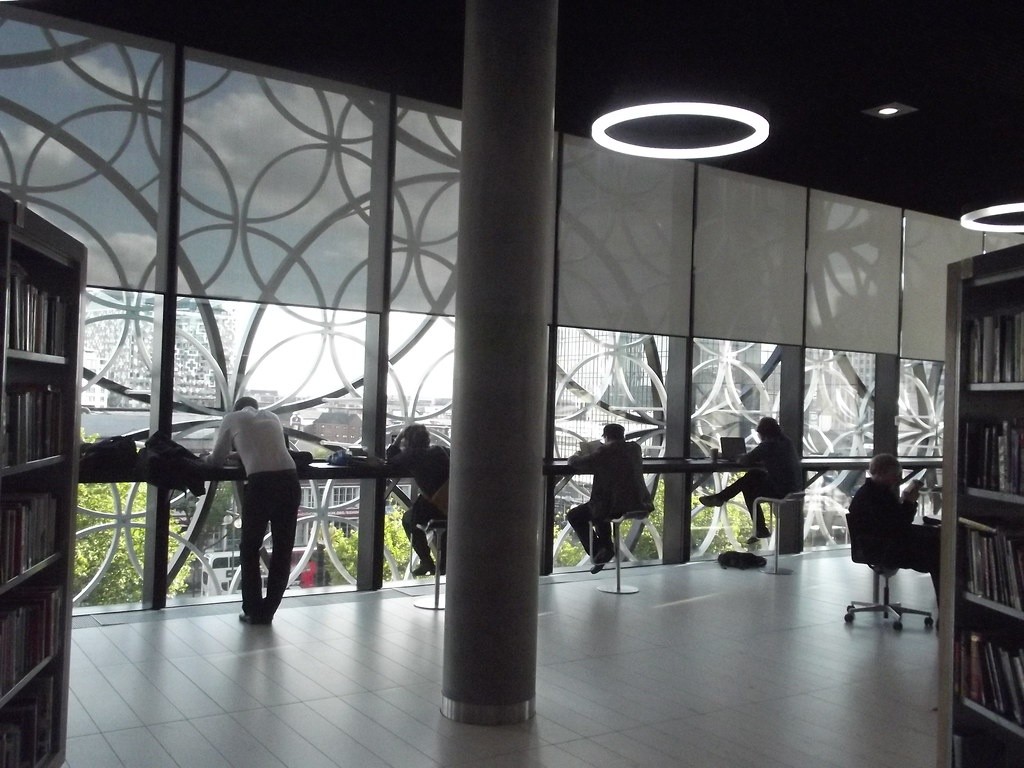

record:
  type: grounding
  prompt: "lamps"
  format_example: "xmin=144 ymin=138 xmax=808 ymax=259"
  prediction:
xmin=961 ymin=202 xmax=1024 ymax=233
xmin=591 ymin=101 xmax=769 ymax=160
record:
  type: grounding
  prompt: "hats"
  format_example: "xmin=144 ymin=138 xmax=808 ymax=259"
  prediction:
xmin=602 ymin=424 xmax=624 ymax=435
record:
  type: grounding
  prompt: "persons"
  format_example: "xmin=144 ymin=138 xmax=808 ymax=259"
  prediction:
xmin=566 ymin=424 xmax=655 ymax=574
xmin=848 ymin=453 xmax=981 ymax=630
xmin=198 ymin=397 xmax=302 ymax=625
xmin=699 ymin=417 xmax=803 ymax=545
xmin=383 ymin=424 xmax=451 ymax=576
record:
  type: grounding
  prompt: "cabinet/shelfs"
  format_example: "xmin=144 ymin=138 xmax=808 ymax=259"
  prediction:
xmin=937 ymin=243 xmax=1024 ymax=768
xmin=0 ymin=189 xmax=88 ymax=768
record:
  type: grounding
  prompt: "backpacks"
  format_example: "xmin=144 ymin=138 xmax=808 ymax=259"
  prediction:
xmin=79 ymin=436 xmax=138 ymax=482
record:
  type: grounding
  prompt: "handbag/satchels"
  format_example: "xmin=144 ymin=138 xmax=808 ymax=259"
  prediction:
xmin=429 ymin=478 xmax=448 ymax=516
xmin=718 ymin=551 xmax=767 ymax=569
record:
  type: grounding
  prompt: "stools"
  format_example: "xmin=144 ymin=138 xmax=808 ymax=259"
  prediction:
xmin=590 ymin=510 xmax=649 ymax=594
xmin=749 ymin=492 xmax=805 ymax=576
xmin=413 ymin=519 xmax=449 ymax=609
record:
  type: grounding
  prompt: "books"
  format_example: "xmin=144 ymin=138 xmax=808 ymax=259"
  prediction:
xmin=0 ymin=280 xmax=66 ymax=767
xmin=952 ymin=312 xmax=1024 ymax=768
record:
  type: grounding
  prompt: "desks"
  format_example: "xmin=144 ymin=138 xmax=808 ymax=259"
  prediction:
xmin=204 ymin=455 xmax=945 ymax=496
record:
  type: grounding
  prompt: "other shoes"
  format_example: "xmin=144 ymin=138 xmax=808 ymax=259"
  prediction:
xmin=590 ymin=548 xmax=614 ymax=574
xmin=748 ymin=530 xmax=771 ymax=544
xmin=699 ymin=494 xmax=723 ymax=506
xmin=594 ymin=548 xmax=607 ymax=562
xmin=240 ymin=614 xmax=272 ymax=624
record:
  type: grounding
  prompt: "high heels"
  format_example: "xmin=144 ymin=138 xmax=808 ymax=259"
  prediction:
xmin=413 ymin=560 xmax=435 ymax=575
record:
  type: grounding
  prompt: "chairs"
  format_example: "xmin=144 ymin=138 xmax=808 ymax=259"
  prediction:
xmin=844 ymin=507 xmax=933 ymax=631
